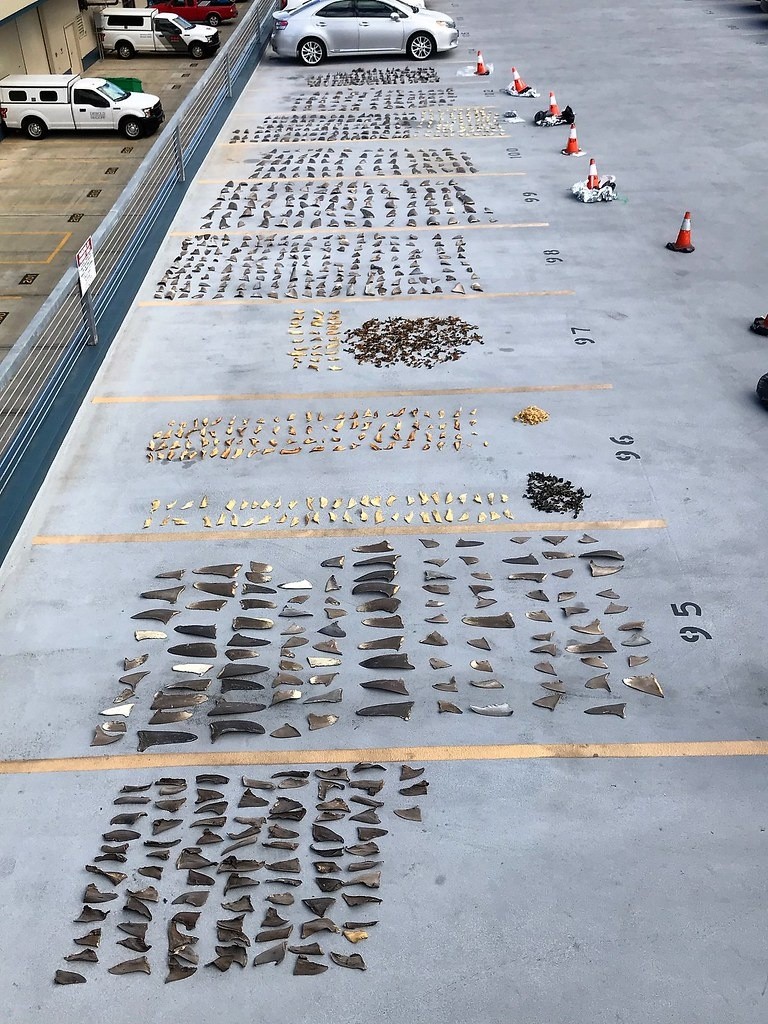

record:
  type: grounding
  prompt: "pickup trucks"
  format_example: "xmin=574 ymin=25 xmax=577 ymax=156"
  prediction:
xmin=146 ymin=0 xmax=239 ymax=27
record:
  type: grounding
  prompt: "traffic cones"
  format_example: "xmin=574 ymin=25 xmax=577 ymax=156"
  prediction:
xmin=548 ymin=91 xmax=561 ymax=116
xmin=512 ymin=67 xmax=532 ymax=94
xmin=561 ymin=123 xmax=582 ymax=156
xmin=586 ymin=158 xmax=599 ymax=190
xmin=474 ymin=51 xmax=490 ymax=76
xmin=665 ymin=211 xmax=695 ymax=253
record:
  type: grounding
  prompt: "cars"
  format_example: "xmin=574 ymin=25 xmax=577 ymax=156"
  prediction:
xmin=271 ymin=0 xmax=461 ymax=67
xmin=280 ymin=0 xmax=427 ymax=10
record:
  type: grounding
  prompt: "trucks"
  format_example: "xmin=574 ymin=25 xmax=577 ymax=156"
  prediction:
xmin=0 ymin=74 xmax=165 ymax=141
xmin=99 ymin=7 xmax=222 ymax=60
